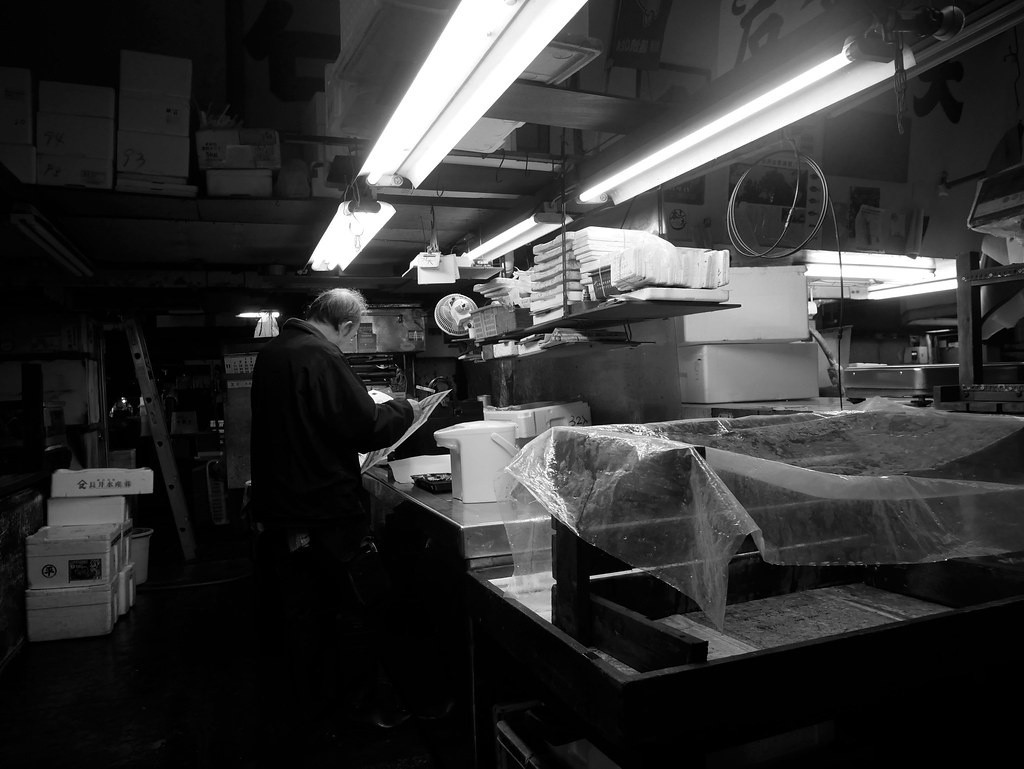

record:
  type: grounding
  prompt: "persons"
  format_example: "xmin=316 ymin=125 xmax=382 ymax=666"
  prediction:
xmin=248 ymin=283 xmax=441 ymax=734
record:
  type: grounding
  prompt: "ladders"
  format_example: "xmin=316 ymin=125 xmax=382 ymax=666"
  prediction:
xmin=126 ymin=318 xmax=207 ymax=565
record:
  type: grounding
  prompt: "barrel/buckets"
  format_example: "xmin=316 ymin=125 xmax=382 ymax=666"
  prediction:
xmin=130 ymin=526 xmax=154 ymax=586
